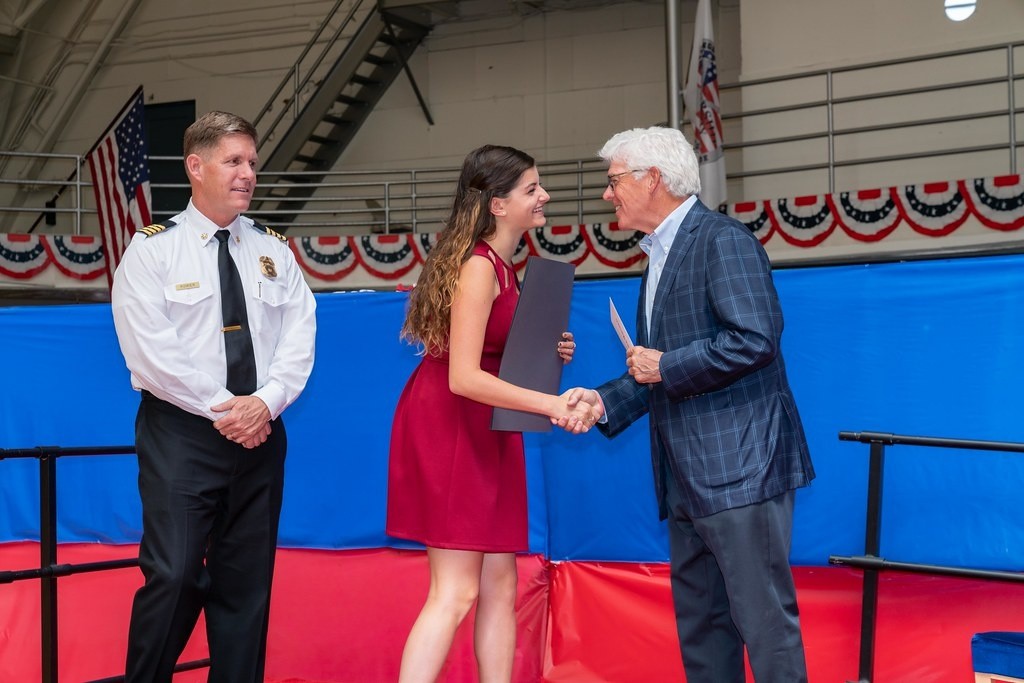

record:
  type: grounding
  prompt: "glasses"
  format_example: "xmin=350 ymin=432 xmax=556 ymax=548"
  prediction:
xmin=608 ymin=170 xmax=639 ymax=191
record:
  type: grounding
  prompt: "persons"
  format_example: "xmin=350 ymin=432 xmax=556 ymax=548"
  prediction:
xmin=112 ymin=111 xmax=313 ymax=683
xmin=552 ymin=125 xmax=814 ymax=683
xmin=386 ymin=144 xmax=601 ymax=683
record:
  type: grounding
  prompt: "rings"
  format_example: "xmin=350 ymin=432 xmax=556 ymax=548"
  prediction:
xmin=589 ymin=416 xmax=595 ymax=422
xmin=230 ymin=433 xmax=237 ymax=440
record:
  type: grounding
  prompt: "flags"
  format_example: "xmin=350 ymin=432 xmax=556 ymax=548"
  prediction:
xmin=86 ymin=92 xmax=152 ymax=300
xmin=681 ymin=0 xmax=728 ymax=211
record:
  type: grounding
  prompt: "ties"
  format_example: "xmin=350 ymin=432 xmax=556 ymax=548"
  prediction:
xmin=214 ymin=230 xmax=257 ymax=397
